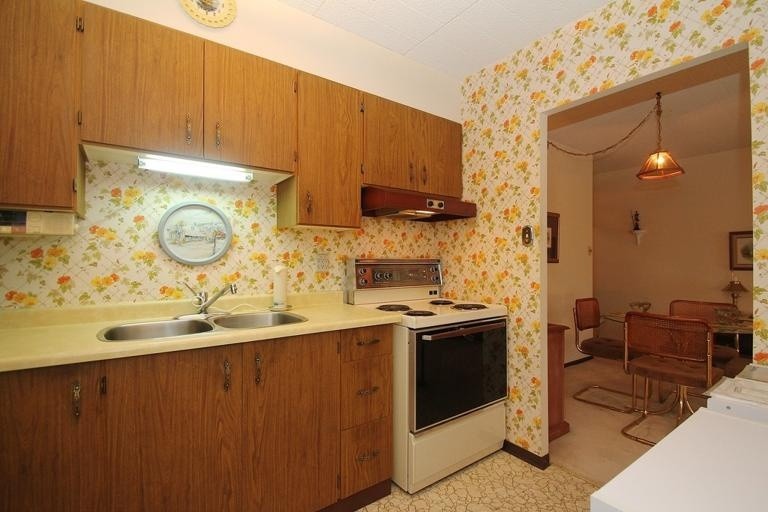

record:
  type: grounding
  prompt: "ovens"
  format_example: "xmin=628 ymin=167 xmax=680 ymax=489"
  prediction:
xmin=407 ymin=317 xmax=509 ymax=484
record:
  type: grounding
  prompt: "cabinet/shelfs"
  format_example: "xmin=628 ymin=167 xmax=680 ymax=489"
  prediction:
xmin=0 ymin=0 xmax=87 ymax=235
xmin=341 ymin=324 xmax=394 ymax=512
xmin=362 ymin=91 xmax=463 ymax=200
xmin=79 ymin=2 xmax=297 ymax=189
xmin=110 ymin=332 xmax=341 ymax=512
xmin=1 ymin=361 xmax=110 ymax=512
xmin=548 ymin=322 xmax=570 ymax=443
xmin=277 ymin=71 xmax=360 ymax=232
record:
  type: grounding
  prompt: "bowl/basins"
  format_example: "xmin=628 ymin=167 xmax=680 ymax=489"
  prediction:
xmin=713 ymin=308 xmax=743 ymax=324
xmin=629 ymin=303 xmax=652 ymax=313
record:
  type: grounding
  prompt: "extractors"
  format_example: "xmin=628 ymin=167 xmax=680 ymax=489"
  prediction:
xmin=362 ymin=188 xmax=477 ymax=222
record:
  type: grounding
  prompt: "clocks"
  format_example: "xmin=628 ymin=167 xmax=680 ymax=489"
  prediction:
xmin=178 ymin=0 xmax=235 ymax=28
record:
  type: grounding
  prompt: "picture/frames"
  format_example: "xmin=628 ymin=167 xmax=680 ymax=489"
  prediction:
xmin=156 ymin=200 xmax=232 ymax=266
xmin=547 ymin=212 xmax=560 ymax=263
xmin=729 ymin=231 xmax=753 ymax=271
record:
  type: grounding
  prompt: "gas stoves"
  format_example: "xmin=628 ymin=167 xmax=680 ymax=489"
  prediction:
xmin=345 ymin=288 xmax=506 ymax=329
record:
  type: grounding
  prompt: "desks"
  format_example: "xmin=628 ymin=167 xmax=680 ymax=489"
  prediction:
xmin=589 ymin=406 xmax=768 ymax=512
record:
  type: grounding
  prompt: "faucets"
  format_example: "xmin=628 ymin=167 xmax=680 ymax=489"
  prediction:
xmin=197 ymin=283 xmax=238 ymax=313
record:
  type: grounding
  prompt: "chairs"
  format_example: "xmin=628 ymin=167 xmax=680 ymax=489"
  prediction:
xmin=573 ymin=297 xmax=753 ymax=447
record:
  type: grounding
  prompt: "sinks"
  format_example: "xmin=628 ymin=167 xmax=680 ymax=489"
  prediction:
xmin=212 ymin=310 xmax=309 ymax=330
xmin=97 ymin=321 xmax=215 ymax=342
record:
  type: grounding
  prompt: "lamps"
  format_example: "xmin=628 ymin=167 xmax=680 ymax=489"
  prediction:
xmin=721 ymin=272 xmax=750 ymax=326
xmin=547 ymin=92 xmax=685 ymax=182
xmin=631 ymin=208 xmax=646 ymax=246
xmin=135 ymin=153 xmax=254 ymax=184
